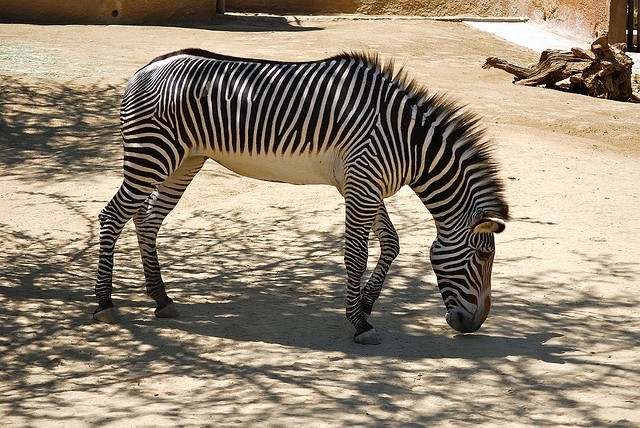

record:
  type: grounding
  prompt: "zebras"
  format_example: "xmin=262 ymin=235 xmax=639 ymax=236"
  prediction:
xmin=92 ymin=47 xmax=515 ymax=346
